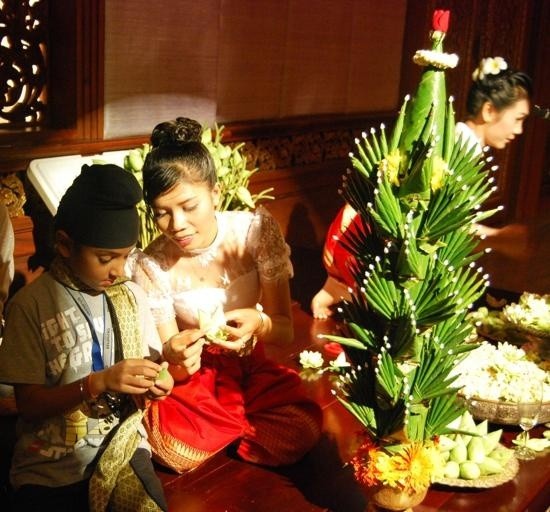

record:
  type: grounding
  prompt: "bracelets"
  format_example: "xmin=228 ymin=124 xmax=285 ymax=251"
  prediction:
xmin=78 ymin=373 xmax=97 ymax=401
xmin=253 ymin=310 xmax=265 ymax=334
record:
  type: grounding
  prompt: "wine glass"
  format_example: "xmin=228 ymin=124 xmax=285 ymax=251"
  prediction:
xmin=513 ymin=380 xmax=543 ymax=462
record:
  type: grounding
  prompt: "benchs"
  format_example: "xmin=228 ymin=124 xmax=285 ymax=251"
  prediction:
xmin=0 ymin=155 xmax=550 ymax=510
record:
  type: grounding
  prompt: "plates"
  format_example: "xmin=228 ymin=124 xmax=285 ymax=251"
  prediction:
xmin=420 ymin=437 xmax=520 ymax=492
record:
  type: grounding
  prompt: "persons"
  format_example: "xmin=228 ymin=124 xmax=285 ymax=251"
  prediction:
xmin=130 ymin=113 xmax=325 ymax=481
xmin=309 ymin=56 xmax=533 ymax=326
xmin=1 ymin=162 xmax=177 ymax=512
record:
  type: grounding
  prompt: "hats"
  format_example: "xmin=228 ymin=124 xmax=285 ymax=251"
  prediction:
xmin=50 ymin=162 xmax=145 ymax=249
